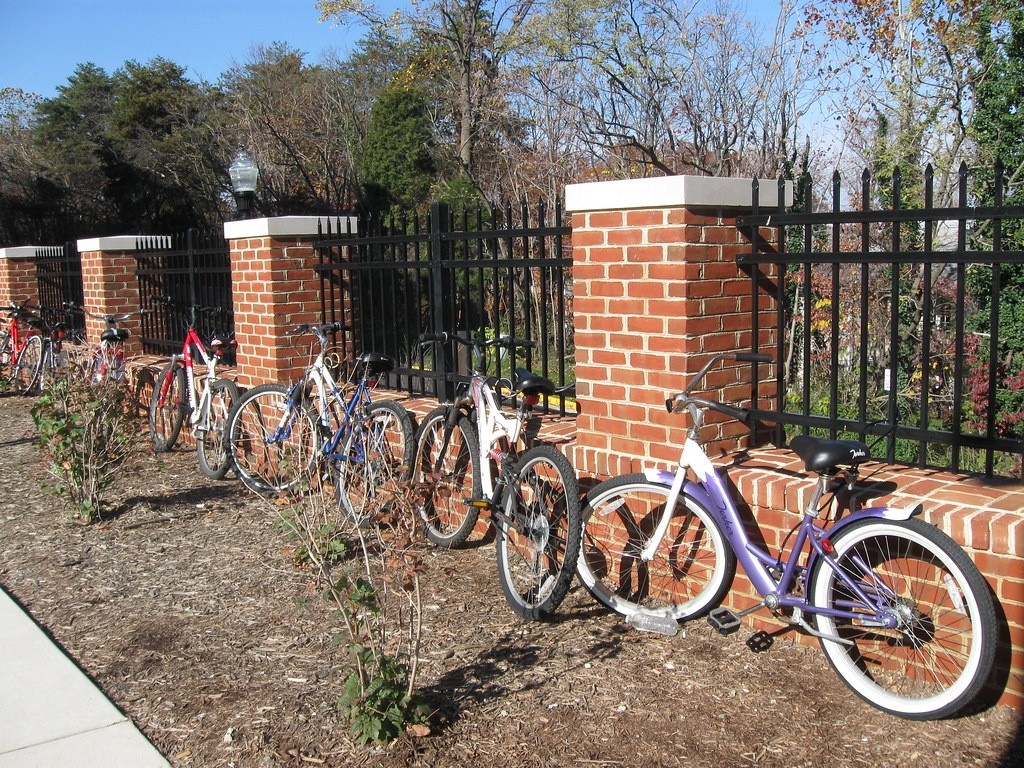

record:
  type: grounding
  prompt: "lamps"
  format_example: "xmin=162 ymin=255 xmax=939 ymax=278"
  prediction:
xmin=230 ymin=149 xmax=259 ymax=220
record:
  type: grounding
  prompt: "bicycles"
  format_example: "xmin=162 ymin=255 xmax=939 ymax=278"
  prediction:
xmin=405 ymin=331 xmax=584 ymax=621
xmin=30 ymin=301 xmax=137 ymax=438
xmin=222 ymin=321 xmax=414 ymax=524
xmin=0 ymin=295 xmax=50 ymax=393
xmin=142 ymin=293 xmax=245 ymax=478
xmin=581 ymin=349 xmax=996 ymax=721
